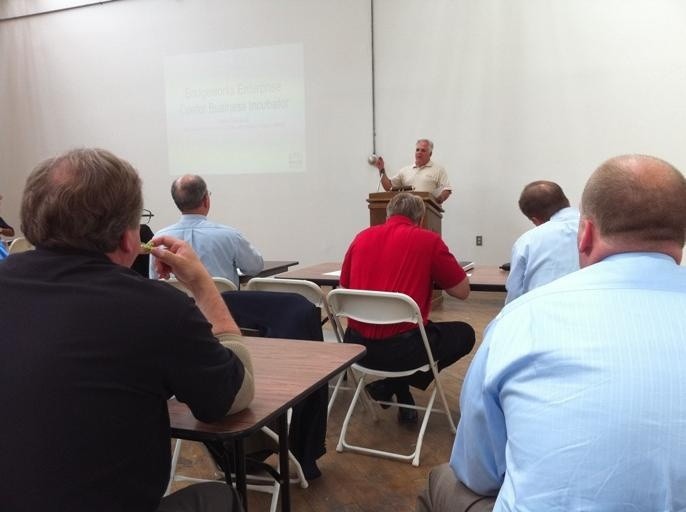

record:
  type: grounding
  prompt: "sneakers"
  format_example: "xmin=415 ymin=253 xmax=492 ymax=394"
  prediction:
xmin=298 ymin=449 xmax=322 ymax=482
xmin=367 ymin=380 xmax=392 ymax=408
xmin=397 ymin=406 xmax=417 ymax=424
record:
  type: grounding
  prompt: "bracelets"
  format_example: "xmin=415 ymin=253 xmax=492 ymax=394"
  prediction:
xmin=379 ymin=168 xmax=385 ymax=176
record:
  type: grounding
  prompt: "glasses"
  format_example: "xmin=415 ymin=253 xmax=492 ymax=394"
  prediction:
xmin=207 ymin=190 xmax=211 ymax=197
xmin=138 ymin=209 xmax=154 ymax=225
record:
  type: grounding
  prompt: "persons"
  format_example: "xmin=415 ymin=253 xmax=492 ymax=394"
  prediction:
xmin=340 ymin=193 xmax=475 ymax=424
xmin=0 ymin=145 xmax=255 ymax=512
xmin=374 ymin=138 xmax=453 ymax=205
xmin=148 ymin=174 xmax=264 ymax=292
xmin=133 ymin=224 xmax=155 ymax=280
xmin=0 ymin=241 xmax=9 ymax=260
xmin=413 ymin=154 xmax=686 ymax=512
xmin=0 ymin=217 xmax=15 ymax=237
xmin=503 ymin=180 xmax=580 ymax=308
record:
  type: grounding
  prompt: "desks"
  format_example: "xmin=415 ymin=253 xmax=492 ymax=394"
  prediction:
xmin=164 ymin=330 xmax=369 ymax=511
xmin=223 ymin=261 xmax=510 ymax=382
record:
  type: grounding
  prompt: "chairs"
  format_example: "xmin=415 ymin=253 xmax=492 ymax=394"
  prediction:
xmin=163 ymin=289 xmax=327 ymax=511
xmin=326 ymin=287 xmax=458 ymax=468
xmin=158 ymin=278 xmax=237 ymax=307
xmin=248 ymin=280 xmax=355 ymax=423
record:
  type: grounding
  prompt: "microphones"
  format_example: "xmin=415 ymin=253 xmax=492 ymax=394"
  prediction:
xmin=377 ymin=168 xmax=385 ymax=192
